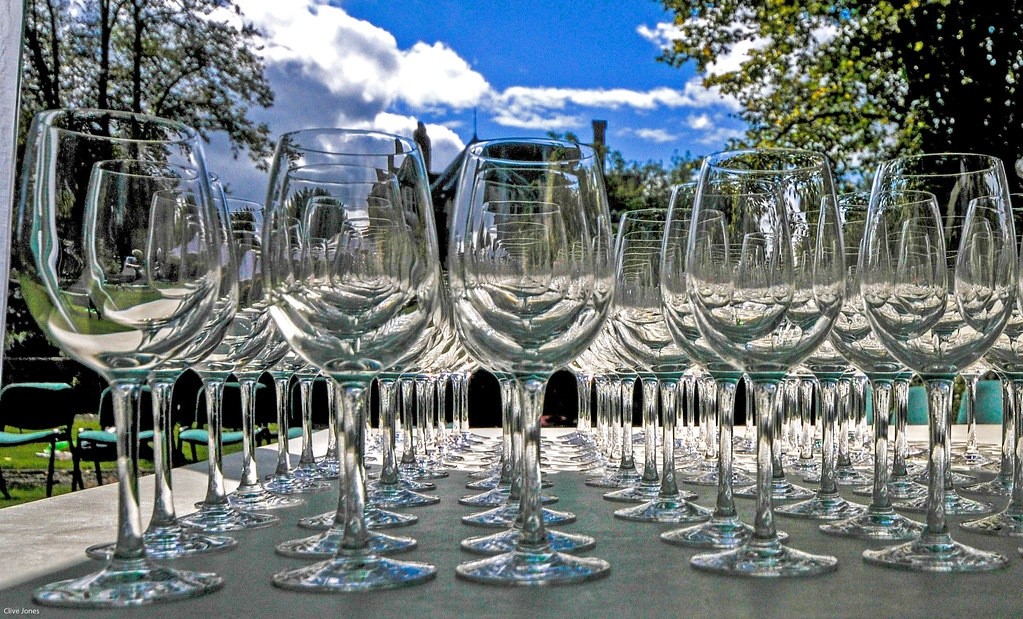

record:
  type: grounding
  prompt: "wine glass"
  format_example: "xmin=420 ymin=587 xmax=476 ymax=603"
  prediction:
xmin=26 ymin=108 xmax=1021 ymax=607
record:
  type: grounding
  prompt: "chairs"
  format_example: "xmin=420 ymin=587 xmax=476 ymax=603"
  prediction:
xmin=176 ymin=382 xmax=270 ymax=470
xmin=0 ymin=383 xmax=84 ymax=497
xmin=72 ymin=382 xmax=174 ymax=491
